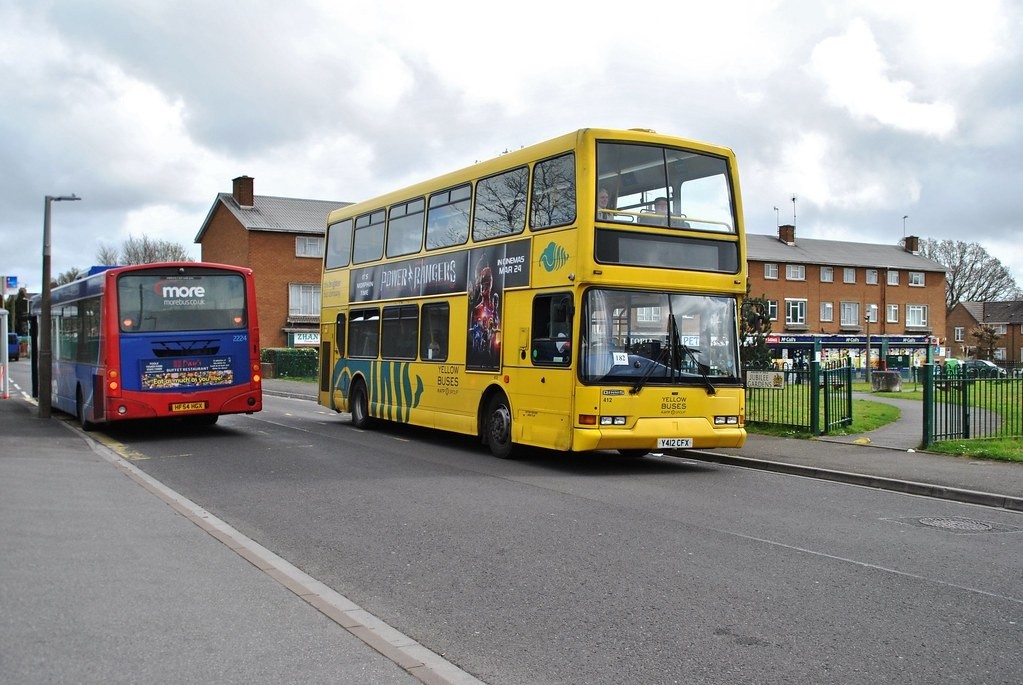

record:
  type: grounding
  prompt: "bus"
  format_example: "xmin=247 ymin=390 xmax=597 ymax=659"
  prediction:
xmin=317 ymin=129 xmax=746 ymax=461
xmin=27 ymin=262 xmax=263 ymax=432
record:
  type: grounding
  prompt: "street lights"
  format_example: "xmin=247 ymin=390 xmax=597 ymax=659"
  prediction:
xmin=863 ymin=310 xmax=871 ymax=383
xmin=38 ymin=192 xmax=83 ymax=419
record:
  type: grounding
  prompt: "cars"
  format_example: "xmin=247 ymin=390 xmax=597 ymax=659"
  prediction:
xmin=965 ymin=359 xmax=1007 ymax=378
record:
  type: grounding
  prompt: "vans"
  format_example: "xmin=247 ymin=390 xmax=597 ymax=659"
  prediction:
xmin=7 ymin=333 xmax=20 ymax=362
